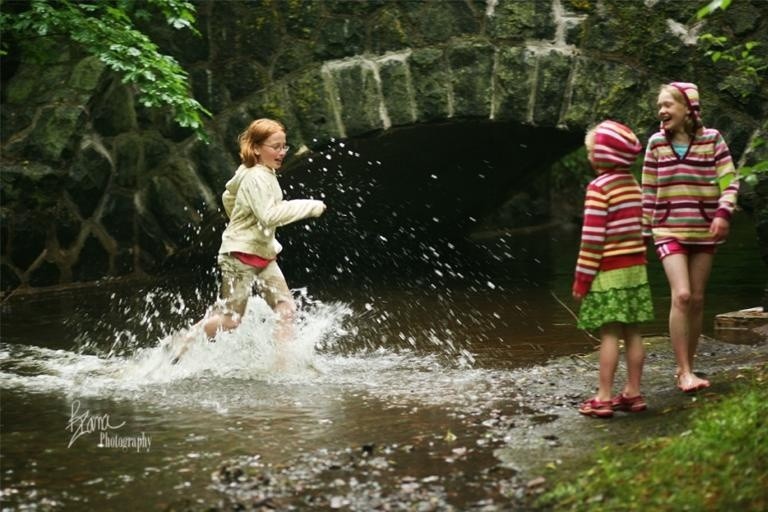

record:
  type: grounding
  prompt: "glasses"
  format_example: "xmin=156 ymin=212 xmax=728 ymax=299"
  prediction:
xmin=263 ymin=144 xmax=290 ymax=152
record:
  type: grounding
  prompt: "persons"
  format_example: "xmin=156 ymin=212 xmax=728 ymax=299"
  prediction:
xmin=638 ymin=82 xmax=741 ymax=393
xmin=571 ymin=117 xmax=654 ymax=420
xmin=204 ymin=117 xmax=326 ymax=336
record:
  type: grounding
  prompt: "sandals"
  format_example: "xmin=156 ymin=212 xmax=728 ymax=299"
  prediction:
xmin=612 ymin=392 xmax=647 ymax=413
xmin=577 ymin=398 xmax=613 ymax=418
